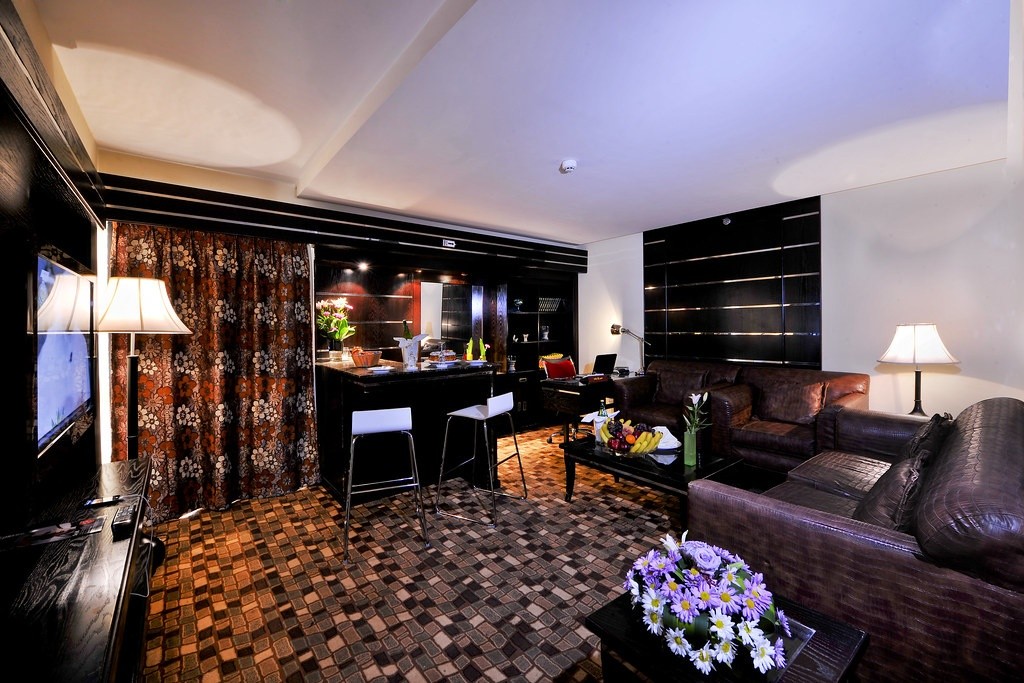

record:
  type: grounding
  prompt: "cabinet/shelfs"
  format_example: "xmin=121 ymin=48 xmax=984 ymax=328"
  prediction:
xmin=506 ymin=311 xmax=579 ymax=433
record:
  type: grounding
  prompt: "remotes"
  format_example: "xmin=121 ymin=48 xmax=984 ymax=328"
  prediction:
xmin=111 ymin=505 xmax=136 ymax=534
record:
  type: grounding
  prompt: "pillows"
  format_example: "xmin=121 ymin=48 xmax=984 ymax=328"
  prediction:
xmin=893 ymin=412 xmax=952 ymax=461
xmin=851 ymin=450 xmax=934 ymax=533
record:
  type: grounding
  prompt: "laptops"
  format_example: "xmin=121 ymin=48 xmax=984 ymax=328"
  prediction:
xmin=577 ymin=354 xmax=617 ymax=377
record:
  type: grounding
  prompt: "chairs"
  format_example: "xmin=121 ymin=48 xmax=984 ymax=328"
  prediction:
xmin=541 ymin=356 xmax=595 ymax=443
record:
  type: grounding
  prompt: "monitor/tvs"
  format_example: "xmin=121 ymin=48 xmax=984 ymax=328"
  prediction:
xmin=36 ymin=252 xmax=92 ymax=448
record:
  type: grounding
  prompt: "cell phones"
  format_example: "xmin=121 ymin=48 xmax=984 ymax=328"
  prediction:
xmin=83 ymin=495 xmax=120 ymax=507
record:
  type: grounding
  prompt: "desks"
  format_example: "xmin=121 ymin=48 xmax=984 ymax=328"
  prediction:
xmin=583 ymin=579 xmax=867 ymax=683
xmin=559 ymin=435 xmax=744 ymax=525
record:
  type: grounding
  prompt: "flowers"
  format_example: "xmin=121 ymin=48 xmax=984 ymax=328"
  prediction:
xmin=625 ymin=531 xmax=791 ymax=674
xmin=316 ymin=298 xmax=356 ymax=342
xmin=682 ymin=392 xmax=713 ymax=433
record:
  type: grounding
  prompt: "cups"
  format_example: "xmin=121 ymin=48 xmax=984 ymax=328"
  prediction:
xmin=343 ymin=347 xmax=349 ymax=357
xmin=430 ymin=350 xmax=456 ymax=361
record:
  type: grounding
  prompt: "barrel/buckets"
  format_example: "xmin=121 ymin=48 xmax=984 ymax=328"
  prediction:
xmin=400 ymin=341 xmax=418 ymax=370
xmin=594 ymin=418 xmax=614 ymax=443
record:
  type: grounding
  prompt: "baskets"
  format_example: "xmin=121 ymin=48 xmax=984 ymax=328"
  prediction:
xmin=352 ymin=351 xmax=382 ymax=368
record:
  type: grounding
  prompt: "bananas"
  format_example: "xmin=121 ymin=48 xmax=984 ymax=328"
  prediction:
xmin=631 ymin=431 xmax=663 ymax=453
xmin=600 ymin=418 xmax=613 ymax=443
xmin=619 ymin=418 xmax=631 ymax=426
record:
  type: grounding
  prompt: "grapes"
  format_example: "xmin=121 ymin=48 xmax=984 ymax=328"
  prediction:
xmin=607 ymin=419 xmax=622 ymax=436
xmin=634 ymin=423 xmax=655 ymax=436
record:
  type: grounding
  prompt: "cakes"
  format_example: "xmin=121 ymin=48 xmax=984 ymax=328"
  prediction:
xmin=430 ymin=351 xmax=457 ymax=361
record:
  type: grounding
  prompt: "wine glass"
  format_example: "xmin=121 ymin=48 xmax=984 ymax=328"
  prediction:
xmin=438 ymin=342 xmax=445 ymax=362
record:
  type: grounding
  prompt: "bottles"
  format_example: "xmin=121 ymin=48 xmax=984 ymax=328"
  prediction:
xmin=403 ymin=320 xmax=413 ymax=340
xmin=598 ymin=400 xmax=608 ymax=417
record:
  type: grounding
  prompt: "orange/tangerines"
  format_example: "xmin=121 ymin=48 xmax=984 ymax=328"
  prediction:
xmin=626 ymin=435 xmax=635 ymax=443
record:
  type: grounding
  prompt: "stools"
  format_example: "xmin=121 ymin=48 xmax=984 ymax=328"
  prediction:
xmin=435 ymin=391 xmax=527 ymax=526
xmin=342 ymin=407 xmax=431 ymax=564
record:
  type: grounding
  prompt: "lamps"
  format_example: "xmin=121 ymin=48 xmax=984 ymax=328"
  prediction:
xmin=877 ymin=322 xmax=961 ymax=415
xmin=95 ymin=275 xmax=193 ymax=462
xmin=27 ymin=273 xmax=91 ymax=335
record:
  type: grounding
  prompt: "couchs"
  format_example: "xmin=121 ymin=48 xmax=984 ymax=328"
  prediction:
xmin=607 ymin=360 xmax=740 ymax=437
xmin=687 ymin=397 xmax=1024 ymax=683
xmin=706 ymin=366 xmax=870 ymax=474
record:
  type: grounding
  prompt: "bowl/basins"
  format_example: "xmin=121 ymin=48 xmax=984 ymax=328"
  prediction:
xmin=330 ymin=351 xmax=342 ymax=368
xmin=429 ymin=361 xmax=460 ymax=368
xmin=352 ymin=351 xmax=382 ymax=367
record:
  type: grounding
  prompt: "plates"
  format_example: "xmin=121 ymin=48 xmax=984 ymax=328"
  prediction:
xmin=465 ymin=360 xmax=487 ymax=366
xmin=368 ymin=367 xmax=394 ymax=374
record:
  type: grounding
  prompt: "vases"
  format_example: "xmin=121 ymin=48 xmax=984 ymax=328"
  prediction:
xmin=328 ymin=337 xmax=343 ymax=351
xmin=684 ymin=429 xmax=700 ymax=466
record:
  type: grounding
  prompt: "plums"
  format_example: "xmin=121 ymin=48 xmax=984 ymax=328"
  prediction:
xmin=608 ymin=431 xmax=640 ymax=453
